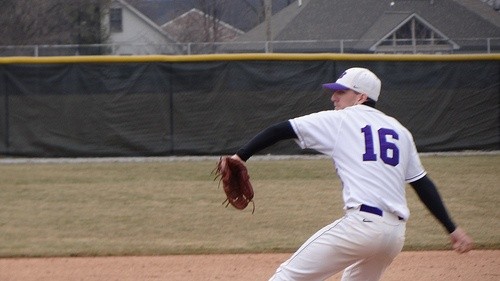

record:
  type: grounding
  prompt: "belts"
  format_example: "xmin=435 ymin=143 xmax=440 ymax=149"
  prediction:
xmin=359 ymin=205 xmax=406 ymax=220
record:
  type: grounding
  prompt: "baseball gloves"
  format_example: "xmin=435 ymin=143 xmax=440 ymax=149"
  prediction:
xmin=209 ymin=155 xmax=255 ymax=215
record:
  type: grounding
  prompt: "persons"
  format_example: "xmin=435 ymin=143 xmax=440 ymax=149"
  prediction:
xmin=216 ymin=67 xmax=475 ymax=281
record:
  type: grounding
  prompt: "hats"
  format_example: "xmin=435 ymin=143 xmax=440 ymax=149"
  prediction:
xmin=321 ymin=67 xmax=382 ymax=102
